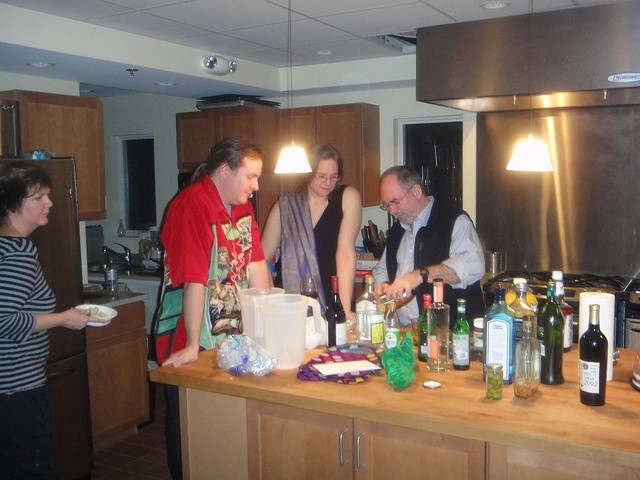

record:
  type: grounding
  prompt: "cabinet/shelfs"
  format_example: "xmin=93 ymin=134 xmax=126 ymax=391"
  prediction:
xmin=175 ymin=108 xmax=258 ymax=172
xmin=487 ymin=428 xmax=640 ymax=480
xmin=0 ymin=90 xmax=108 ymax=222
xmin=280 ymin=104 xmax=382 ymax=208
xmin=259 ymin=388 xmax=487 ymax=479
xmin=85 ymin=297 xmax=151 ymax=453
xmin=256 ymin=106 xmax=279 ymax=240
xmin=175 ymin=376 xmax=259 ymax=479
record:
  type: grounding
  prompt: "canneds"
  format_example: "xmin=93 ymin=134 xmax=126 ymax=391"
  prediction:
xmin=484 ymin=360 xmax=504 ymax=402
xmin=472 ymin=317 xmax=485 ymax=354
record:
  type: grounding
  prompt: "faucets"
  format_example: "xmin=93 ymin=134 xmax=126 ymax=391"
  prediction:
xmin=98 ymin=241 xmax=134 ymax=264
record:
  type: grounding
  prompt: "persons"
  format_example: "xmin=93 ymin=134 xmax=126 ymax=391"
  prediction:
xmin=0 ymin=162 xmax=106 ymax=477
xmin=153 ymin=136 xmax=264 ymax=480
xmin=370 ymin=166 xmax=485 ymax=328
xmin=261 ymin=143 xmax=363 ymax=324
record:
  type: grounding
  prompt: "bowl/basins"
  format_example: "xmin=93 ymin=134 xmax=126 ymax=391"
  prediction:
xmin=73 ymin=302 xmax=119 ymax=328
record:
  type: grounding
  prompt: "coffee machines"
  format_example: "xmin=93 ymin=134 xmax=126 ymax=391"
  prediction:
xmin=150 ymin=228 xmax=161 ymax=264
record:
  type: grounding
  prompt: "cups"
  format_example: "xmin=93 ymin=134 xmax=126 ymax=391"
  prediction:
xmin=357 ymin=311 xmax=383 ymax=348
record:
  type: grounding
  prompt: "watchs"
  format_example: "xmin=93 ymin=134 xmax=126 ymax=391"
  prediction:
xmin=418 ymin=266 xmax=428 ymax=284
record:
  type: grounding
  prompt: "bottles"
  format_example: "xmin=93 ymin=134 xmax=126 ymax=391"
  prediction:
xmin=537 ymin=282 xmax=564 ymax=385
xmin=580 ymin=304 xmax=608 ymax=406
xmin=105 ymin=258 xmax=119 ymax=294
xmin=102 ymin=246 xmax=109 ymax=280
xmin=510 ymin=279 xmax=533 ymax=316
xmin=356 ymin=275 xmax=382 ymax=311
xmin=417 ymin=295 xmax=437 ymax=361
xmin=383 ymin=303 xmax=400 ymax=351
xmin=472 ymin=318 xmax=486 ymax=360
xmin=426 ymin=278 xmax=452 ymax=373
xmin=514 ymin=316 xmax=542 ymax=397
xmin=551 ymin=270 xmax=574 ymax=352
xmin=326 ymin=275 xmax=347 ymax=347
xmin=451 ymin=299 xmax=470 ymax=371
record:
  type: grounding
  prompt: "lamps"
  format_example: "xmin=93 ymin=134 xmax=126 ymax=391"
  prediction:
xmin=506 ymin=2 xmax=554 ymax=172
xmin=199 ymin=54 xmax=237 ymax=76
xmin=274 ymin=0 xmax=314 ymax=173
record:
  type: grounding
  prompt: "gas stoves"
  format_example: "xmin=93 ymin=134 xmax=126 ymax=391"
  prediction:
xmin=490 ymin=269 xmax=629 ymax=293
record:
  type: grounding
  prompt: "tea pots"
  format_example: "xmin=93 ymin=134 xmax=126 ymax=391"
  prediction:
xmin=238 ymin=289 xmax=286 ymax=347
xmin=259 ymin=294 xmax=321 ymax=370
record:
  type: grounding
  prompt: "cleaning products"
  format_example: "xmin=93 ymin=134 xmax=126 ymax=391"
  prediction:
xmin=138 ymin=233 xmax=151 ymax=265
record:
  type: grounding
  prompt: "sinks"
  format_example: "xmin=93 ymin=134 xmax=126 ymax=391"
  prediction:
xmin=86 ymin=257 xmax=143 ymax=277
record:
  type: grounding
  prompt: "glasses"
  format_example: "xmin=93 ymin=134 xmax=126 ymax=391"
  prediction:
xmin=315 ymin=175 xmax=341 ymax=182
xmin=380 ymin=182 xmax=421 ymax=211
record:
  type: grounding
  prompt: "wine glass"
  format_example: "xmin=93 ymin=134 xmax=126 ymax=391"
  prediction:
xmin=299 ymin=276 xmax=319 ymax=300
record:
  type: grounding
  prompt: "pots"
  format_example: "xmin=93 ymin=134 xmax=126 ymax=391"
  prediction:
xmin=485 ymin=251 xmax=507 ymax=272
